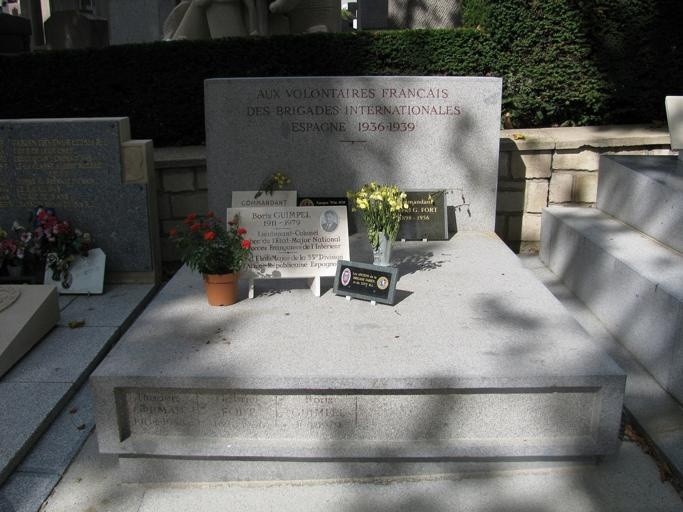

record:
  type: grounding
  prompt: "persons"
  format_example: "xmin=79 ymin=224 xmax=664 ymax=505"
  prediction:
xmin=321 ymin=211 xmax=337 ymax=232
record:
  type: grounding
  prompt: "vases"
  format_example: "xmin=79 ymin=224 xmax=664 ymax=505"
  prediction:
xmin=370 ymin=232 xmax=394 ymax=266
xmin=204 ymin=273 xmax=240 ymax=306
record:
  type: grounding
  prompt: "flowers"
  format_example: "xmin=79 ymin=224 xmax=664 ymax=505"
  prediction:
xmin=345 ymin=179 xmax=413 ymax=250
xmin=168 ymin=211 xmax=251 ymax=279
